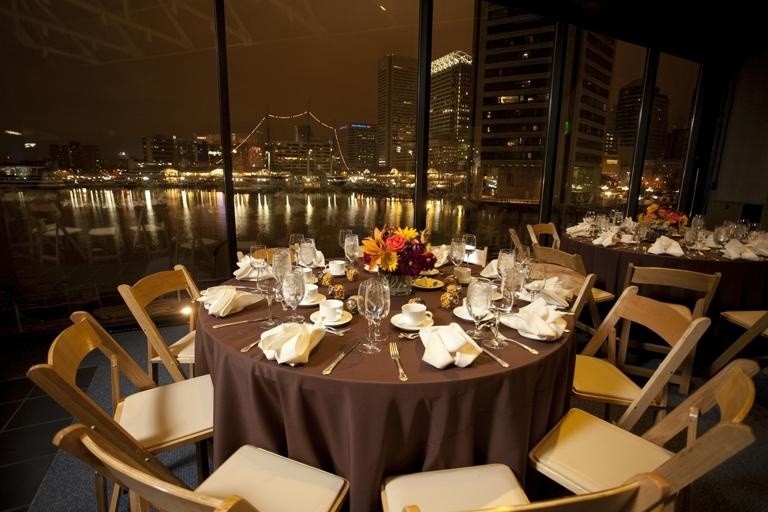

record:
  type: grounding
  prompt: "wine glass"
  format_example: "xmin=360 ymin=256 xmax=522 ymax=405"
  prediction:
xmin=345 ymin=235 xmax=359 ymax=270
xmin=357 ymin=281 xmax=384 ymax=354
xmin=272 ymin=248 xmax=293 ymax=302
xmin=283 ymin=267 xmax=306 ymax=324
xmin=584 ymin=211 xmax=595 ymax=236
xmin=483 ymin=286 xmax=514 ymax=350
xmin=449 ymin=236 xmax=465 ymax=268
xmin=338 ymin=229 xmax=352 ymax=260
xmin=514 ymin=246 xmax=530 ymax=279
xmin=610 ymin=209 xmax=624 ymax=226
xmin=522 ymin=264 xmax=546 ymax=304
xmin=495 ymin=249 xmax=515 ymax=311
xmin=249 ymin=245 xmax=268 ymax=294
xmin=596 ymin=213 xmax=606 ymax=236
xmin=713 ymin=218 xmax=758 ymax=247
xmin=365 ymin=278 xmax=390 ymax=342
xmin=289 ymin=234 xmax=305 ymax=269
xmin=684 ymin=215 xmax=704 ymax=258
xmin=463 ymin=234 xmax=476 ymax=268
xmin=256 ymin=267 xmax=282 ymax=329
xmin=598 ymin=218 xmax=610 ymax=234
xmin=466 ymin=281 xmax=494 ymax=340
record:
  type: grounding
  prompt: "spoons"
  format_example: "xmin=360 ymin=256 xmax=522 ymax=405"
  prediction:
xmin=399 ymin=331 xmax=420 ymax=341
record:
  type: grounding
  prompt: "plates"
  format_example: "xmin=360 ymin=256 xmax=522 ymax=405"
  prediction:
xmin=297 ymin=293 xmax=327 ymax=308
xmin=391 ymin=313 xmax=434 ymax=332
xmin=310 ymin=310 xmax=353 ymax=327
xmin=411 ymin=278 xmax=445 ymax=290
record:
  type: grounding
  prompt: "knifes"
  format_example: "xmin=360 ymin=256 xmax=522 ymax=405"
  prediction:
xmin=212 ymin=315 xmax=279 ymax=329
xmin=321 ymin=341 xmax=360 ymax=375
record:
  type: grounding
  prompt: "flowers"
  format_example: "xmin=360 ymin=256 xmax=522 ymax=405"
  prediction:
xmin=638 ymin=202 xmax=690 ymax=235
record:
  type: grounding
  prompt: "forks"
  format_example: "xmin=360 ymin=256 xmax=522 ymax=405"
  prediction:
xmin=489 ymin=326 xmax=539 ymax=355
xmin=388 ymin=341 xmax=408 ymax=382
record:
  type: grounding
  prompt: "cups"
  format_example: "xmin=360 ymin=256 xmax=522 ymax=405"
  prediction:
xmin=453 ymin=268 xmax=472 ymax=281
xmin=303 ymin=269 xmax=315 ymax=283
xmin=300 ymin=238 xmax=316 ymax=268
xmin=320 ymin=299 xmax=344 ymax=320
xmin=301 ymin=283 xmax=318 ymax=304
xmin=324 ymin=260 xmax=346 ymax=276
xmin=401 ymin=303 xmax=433 ymax=326
